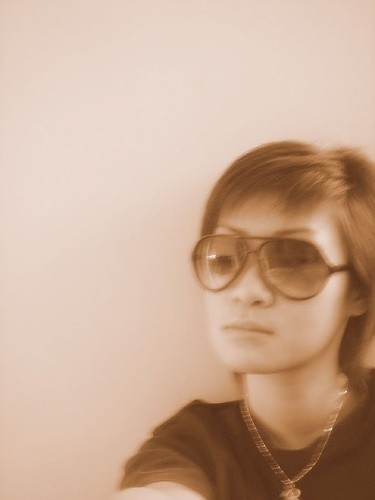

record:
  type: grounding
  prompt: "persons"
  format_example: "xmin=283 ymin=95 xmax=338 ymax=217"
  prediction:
xmin=116 ymin=141 xmax=375 ymax=500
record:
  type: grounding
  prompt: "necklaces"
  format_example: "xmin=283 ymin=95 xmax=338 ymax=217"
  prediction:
xmin=241 ymin=374 xmax=348 ymax=500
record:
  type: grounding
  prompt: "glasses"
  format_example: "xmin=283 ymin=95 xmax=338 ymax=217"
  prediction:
xmin=193 ymin=236 xmax=356 ymax=300
